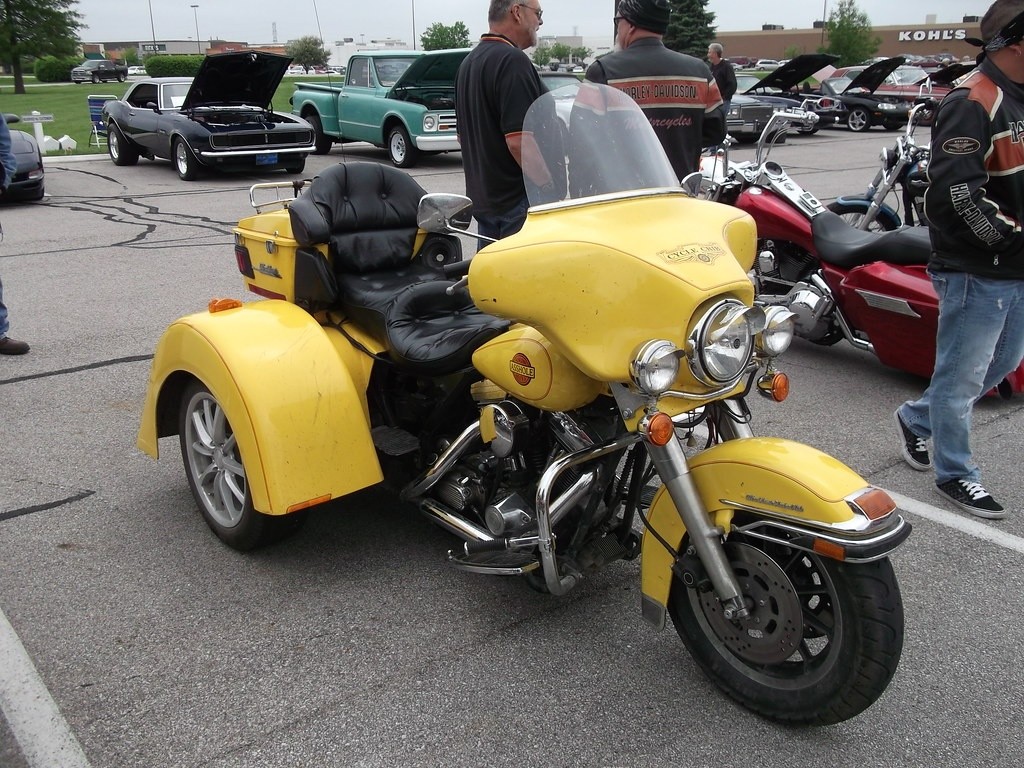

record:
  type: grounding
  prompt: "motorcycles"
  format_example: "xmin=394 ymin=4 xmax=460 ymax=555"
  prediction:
xmin=134 ymin=78 xmax=912 ymax=728
xmin=689 ymin=91 xmax=1024 ymax=402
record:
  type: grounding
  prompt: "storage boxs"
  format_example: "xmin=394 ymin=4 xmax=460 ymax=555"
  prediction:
xmin=234 ymin=211 xmax=429 ymax=305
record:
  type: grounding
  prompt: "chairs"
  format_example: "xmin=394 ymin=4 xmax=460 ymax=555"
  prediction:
xmin=803 ymin=81 xmax=810 ymax=93
xmin=87 ymin=94 xmax=117 ymax=149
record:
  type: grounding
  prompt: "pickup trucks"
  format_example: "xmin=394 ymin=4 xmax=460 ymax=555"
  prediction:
xmin=71 ymin=60 xmax=130 ymax=84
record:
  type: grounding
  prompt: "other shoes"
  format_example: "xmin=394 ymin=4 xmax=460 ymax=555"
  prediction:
xmin=0 ymin=337 xmax=30 ymax=354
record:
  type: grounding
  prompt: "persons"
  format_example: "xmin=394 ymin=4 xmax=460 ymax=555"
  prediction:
xmin=705 ymin=43 xmax=737 ymax=156
xmin=0 ymin=110 xmax=31 ymax=355
xmin=893 ymin=0 xmax=1024 ymax=518
xmin=568 ymin=0 xmax=728 ymax=200
xmin=454 ymin=0 xmax=567 ymax=252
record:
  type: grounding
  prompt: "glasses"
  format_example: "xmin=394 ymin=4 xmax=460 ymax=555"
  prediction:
xmin=510 ymin=4 xmax=543 ymax=20
xmin=614 ymin=16 xmax=626 ymax=27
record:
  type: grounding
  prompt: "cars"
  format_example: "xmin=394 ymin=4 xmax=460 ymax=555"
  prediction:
xmin=128 ymin=66 xmax=148 ymax=75
xmin=101 ymin=51 xmax=316 ymax=183
xmin=0 ymin=113 xmax=47 ymax=201
xmin=291 ymin=47 xmax=475 ymax=167
xmin=698 ymin=52 xmax=981 ymax=144
xmin=281 ymin=64 xmax=346 ymax=75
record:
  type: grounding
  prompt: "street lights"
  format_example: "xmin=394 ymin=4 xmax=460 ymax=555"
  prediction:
xmin=190 ymin=5 xmax=202 ymax=56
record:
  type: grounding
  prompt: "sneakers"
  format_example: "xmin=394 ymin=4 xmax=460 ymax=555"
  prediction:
xmin=893 ymin=410 xmax=931 ymax=471
xmin=932 ymin=477 xmax=1005 ymax=519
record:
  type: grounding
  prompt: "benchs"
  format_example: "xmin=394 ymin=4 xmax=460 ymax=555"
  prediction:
xmin=135 ymin=97 xmax=157 ymax=108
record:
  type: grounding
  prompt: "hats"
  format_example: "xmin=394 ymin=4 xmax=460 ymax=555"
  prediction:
xmin=618 ymin=0 xmax=670 ymax=34
